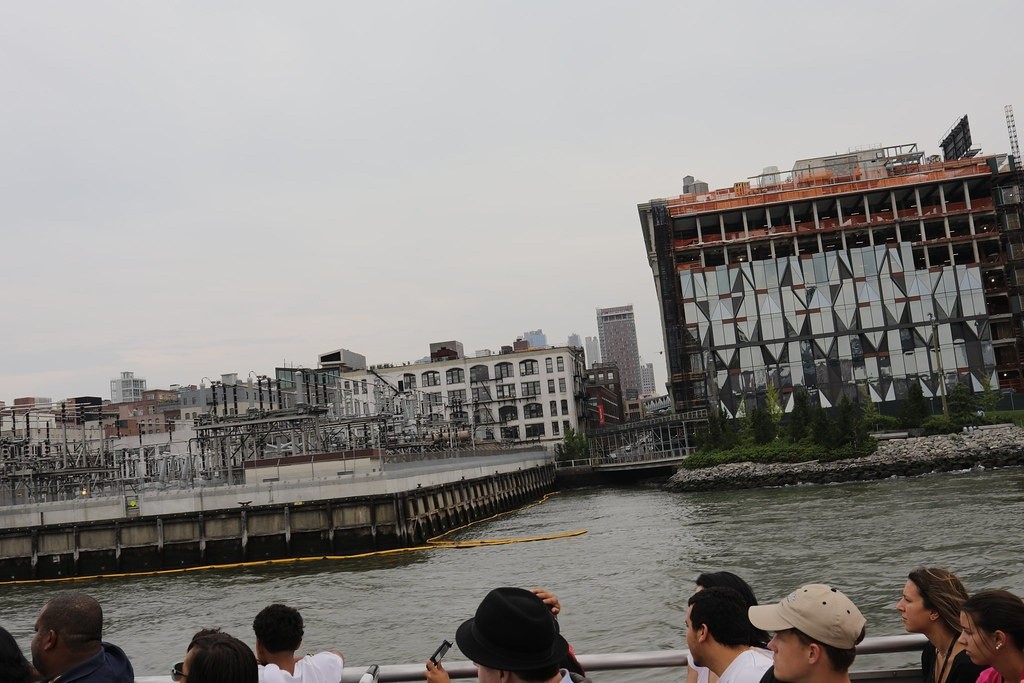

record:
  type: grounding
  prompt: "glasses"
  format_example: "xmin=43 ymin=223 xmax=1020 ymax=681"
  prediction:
xmin=171 ymin=662 xmax=188 ymax=681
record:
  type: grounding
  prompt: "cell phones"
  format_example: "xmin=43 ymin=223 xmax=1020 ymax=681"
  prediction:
xmin=426 ymin=640 xmax=453 ymax=672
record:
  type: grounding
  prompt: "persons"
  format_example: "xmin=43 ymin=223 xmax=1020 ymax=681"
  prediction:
xmin=897 ymin=567 xmax=990 ymax=683
xmin=685 ymin=571 xmax=774 ymax=683
xmin=958 ymin=590 xmax=1024 ymax=683
xmin=181 ymin=627 xmax=259 ymax=683
xmin=424 ymin=589 xmax=592 ymax=683
xmin=0 ymin=627 xmax=40 ymax=683
xmin=253 ymin=604 xmax=344 ymax=683
xmin=748 ymin=584 xmax=867 ymax=683
xmin=30 ymin=592 xmax=134 ymax=683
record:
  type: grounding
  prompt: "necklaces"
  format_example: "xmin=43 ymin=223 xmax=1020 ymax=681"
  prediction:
xmin=929 ymin=635 xmax=960 ymax=683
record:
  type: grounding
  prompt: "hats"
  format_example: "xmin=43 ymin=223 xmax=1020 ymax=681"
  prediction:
xmin=747 ymin=584 xmax=867 ymax=650
xmin=455 ymin=587 xmax=569 ymax=672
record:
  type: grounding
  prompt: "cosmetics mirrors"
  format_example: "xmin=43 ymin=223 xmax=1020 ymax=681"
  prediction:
xmin=426 ymin=640 xmax=453 ymax=671
